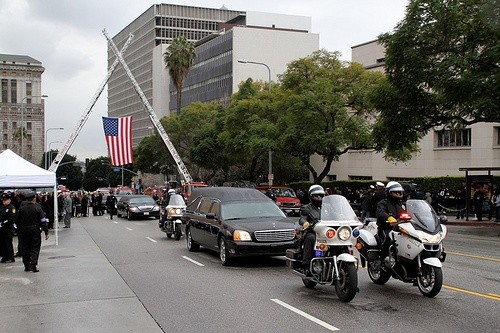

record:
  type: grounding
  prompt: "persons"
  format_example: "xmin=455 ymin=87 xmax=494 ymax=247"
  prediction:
xmin=0 ymin=190 xmax=178 ymax=272
xmin=265 ymin=179 xmax=500 ymax=292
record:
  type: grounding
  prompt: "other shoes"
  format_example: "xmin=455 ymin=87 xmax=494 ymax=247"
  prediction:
xmin=2 ymin=258 xmax=15 ymax=263
xmin=32 ymin=269 xmax=39 ymax=272
xmin=64 ymin=226 xmax=68 ymax=228
xmin=25 ymin=267 xmax=37 ymax=271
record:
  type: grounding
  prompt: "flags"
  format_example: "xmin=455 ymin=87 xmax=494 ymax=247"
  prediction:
xmin=102 ymin=116 xmax=134 ymax=167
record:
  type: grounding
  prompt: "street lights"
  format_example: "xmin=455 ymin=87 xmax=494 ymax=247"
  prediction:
xmin=44 ymin=127 xmax=65 ymax=170
xmin=236 ymin=59 xmax=273 ymax=185
xmin=21 ymin=94 xmax=48 ymax=158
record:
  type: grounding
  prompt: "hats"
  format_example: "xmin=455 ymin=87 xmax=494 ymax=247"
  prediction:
xmin=376 ymin=181 xmax=385 ymax=187
xmin=109 ymin=191 xmax=113 ymax=194
xmin=370 ymin=184 xmax=375 ymax=189
xmin=1 ymin=193 xmax=10 ymax=200
xmin=25 ymin=192 xmax=35 ymax=199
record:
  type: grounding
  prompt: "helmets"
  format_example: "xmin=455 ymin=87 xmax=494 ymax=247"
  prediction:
xmin=385 ymin=181 xmax=404 ymax=193
xmin=309 ymin=185 xmax=325 ymax=197
xmin=168 ymin=189 xmax=175 ymax=195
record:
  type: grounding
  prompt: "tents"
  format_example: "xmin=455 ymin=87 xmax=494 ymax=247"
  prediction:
xmin=0 ymin=149 xmax=58 ymax=248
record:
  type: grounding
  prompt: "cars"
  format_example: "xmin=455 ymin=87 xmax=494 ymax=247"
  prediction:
xmin=91 ymin=189 xmax=161 ymax=219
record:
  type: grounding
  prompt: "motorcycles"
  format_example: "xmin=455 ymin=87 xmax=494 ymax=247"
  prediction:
xmin=159 ymin=196 xmax=186 ymax=240
xmin=283 ymin=194 xmax=364 ymax=303
xmin=356 ymin=199 xmax=449 ymax=297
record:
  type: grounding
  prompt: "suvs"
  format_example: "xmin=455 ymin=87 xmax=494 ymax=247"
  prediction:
xmin=180 ymin=186 xmax=305 ymax=266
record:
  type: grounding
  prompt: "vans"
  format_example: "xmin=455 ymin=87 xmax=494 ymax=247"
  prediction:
xmin=254 ymin=186 xmax=302 ymax=216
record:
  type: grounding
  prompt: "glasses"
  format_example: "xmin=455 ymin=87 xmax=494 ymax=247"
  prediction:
xmin=315 ymin=194 xmax=324 ymax=197
